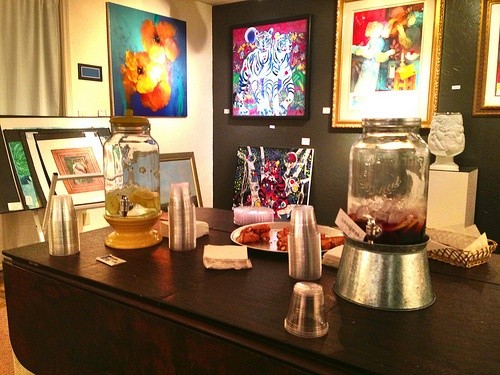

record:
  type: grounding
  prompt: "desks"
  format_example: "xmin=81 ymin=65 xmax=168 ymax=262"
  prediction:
xmin=2 ymin=207 xmax=499 ymax=375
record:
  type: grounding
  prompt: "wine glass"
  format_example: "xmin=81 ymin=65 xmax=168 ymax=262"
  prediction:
xmin=428 ymin=113 xmax=466 ymax=171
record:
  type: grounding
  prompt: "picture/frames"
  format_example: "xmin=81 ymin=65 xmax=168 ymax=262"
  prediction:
xmin=106 ymin=1 xmax=188 ymax=119
xmin=332 ymin=0 xmax=445 ymax=130
xmin=471 ymin=0 xmax=500 ymax=119
xmin=124 ymin=152 xmax=203 ymax=210
xmin=228 ymin=12 xmax=310 ymax=121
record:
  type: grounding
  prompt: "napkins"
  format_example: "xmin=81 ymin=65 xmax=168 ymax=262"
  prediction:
xmin=203 ymin=244 xmax=253 ymax=272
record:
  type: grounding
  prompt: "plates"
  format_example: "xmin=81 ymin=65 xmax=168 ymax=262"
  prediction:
xmin=230 ymin=221 xmax=343 ymax=253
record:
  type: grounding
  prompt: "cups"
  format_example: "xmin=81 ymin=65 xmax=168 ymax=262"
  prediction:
xmin=284 ymin=282 xmax=329 ymax=338
xmin=47 ymin=194 xmax=80 ymax=256
xmin=288 ymin=205 xmax=324 ymax=281
xmin=167 ymin=182 xmax=197 ymax=251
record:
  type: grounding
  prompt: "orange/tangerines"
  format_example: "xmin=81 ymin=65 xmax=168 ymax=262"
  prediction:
xmin=105 ymin=196 xmax=120 ymax=215
xmin=133 ymin=190 xmax=155 ymax=200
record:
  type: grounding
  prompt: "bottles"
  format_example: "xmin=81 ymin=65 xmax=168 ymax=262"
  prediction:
xmin=347 ymin=117 xmax=430 ymax=240
xmin=102 ymin=116 xmax=162 ymax=219
